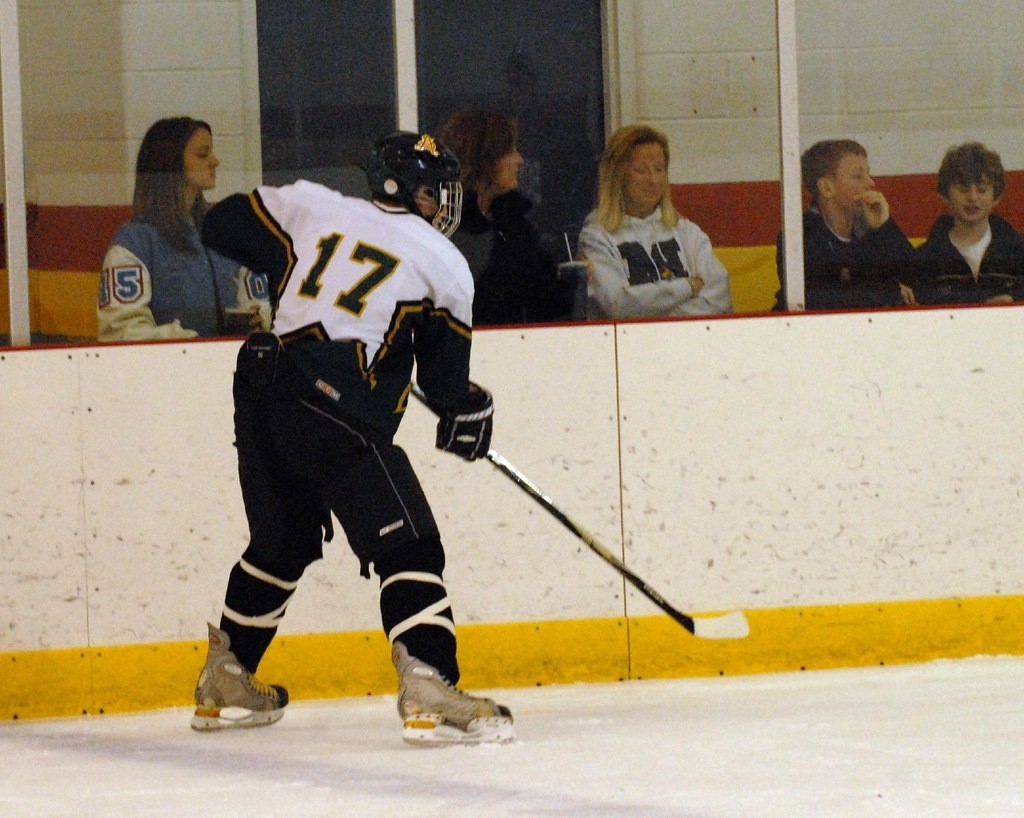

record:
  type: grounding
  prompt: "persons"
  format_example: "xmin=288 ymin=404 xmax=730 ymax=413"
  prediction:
xmin=918 ymin=142 xmax=1024 ymax=303
xmin=96 ymin=116 xmax=272 ymax=342
xmin=574 ymin=125 xmax=731 ymax=320
xmin=772 ymin=139 xmax=934 ymax=311
xmin=438 ymin=108 xmax=584 ymax=328
xmin=190 ymin=130 xmax=518 ymax=747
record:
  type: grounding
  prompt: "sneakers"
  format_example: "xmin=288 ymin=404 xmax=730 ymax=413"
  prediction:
xmin=392 ymin=642 xmax=515 ymax=746
xmin=191 ymin=622 xmax=289 ymax=731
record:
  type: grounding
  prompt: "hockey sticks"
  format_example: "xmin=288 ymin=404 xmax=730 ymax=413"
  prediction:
xmin=410 ymin=380 xmax=750 ymax=640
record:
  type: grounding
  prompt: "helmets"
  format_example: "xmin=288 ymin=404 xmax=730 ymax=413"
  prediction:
xmin=367 ymin=131 xmax=464 ymax=239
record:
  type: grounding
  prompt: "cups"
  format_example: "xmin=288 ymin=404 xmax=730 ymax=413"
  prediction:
xmin=224 ymin=307 xmax=258 ymax=338
xmin=554 ymin=261 xmax=590 ymax=323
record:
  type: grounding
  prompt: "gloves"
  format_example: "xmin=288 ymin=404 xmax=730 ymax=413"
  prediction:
xmin=436 ymin=383 xmax=494 ymax=461
